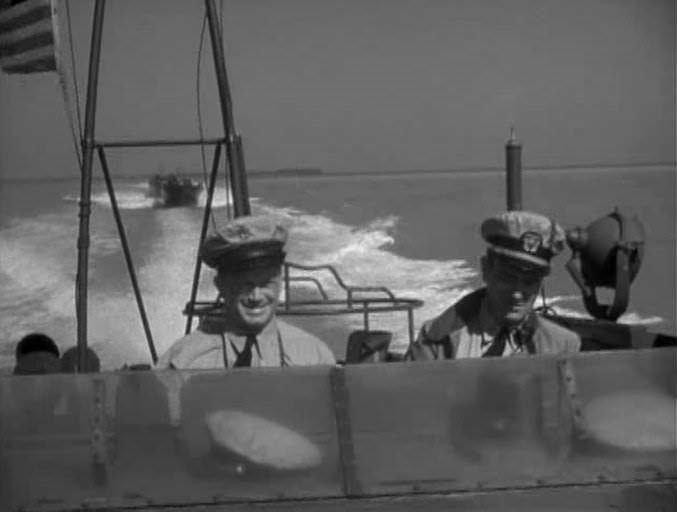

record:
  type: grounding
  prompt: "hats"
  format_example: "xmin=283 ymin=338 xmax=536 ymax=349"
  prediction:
xmin=190 ymin=406 xmax=324 ymax=486
xmin=199 ymin=211 xmax=291 ymax=270
xmin=572 ymin=388 xmax=674 ymax=456
xmin=481 ymin=210 xmax=568 ymax=275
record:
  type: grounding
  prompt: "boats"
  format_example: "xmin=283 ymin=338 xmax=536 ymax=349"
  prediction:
xmin=147 ymin=170 xmax=205 ymax=207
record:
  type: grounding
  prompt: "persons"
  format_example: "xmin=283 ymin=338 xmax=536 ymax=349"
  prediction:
xmin=401 ymin=209 xmax=583 ymax=362
xmin=146 ymin=213 xmax=339 ymax=369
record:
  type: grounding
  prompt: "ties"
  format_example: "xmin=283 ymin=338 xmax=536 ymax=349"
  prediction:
xmin=232 ymin=332 xmax=256 ymax=369
xmin=483 ymin=327 xmax=516 ymax=358
